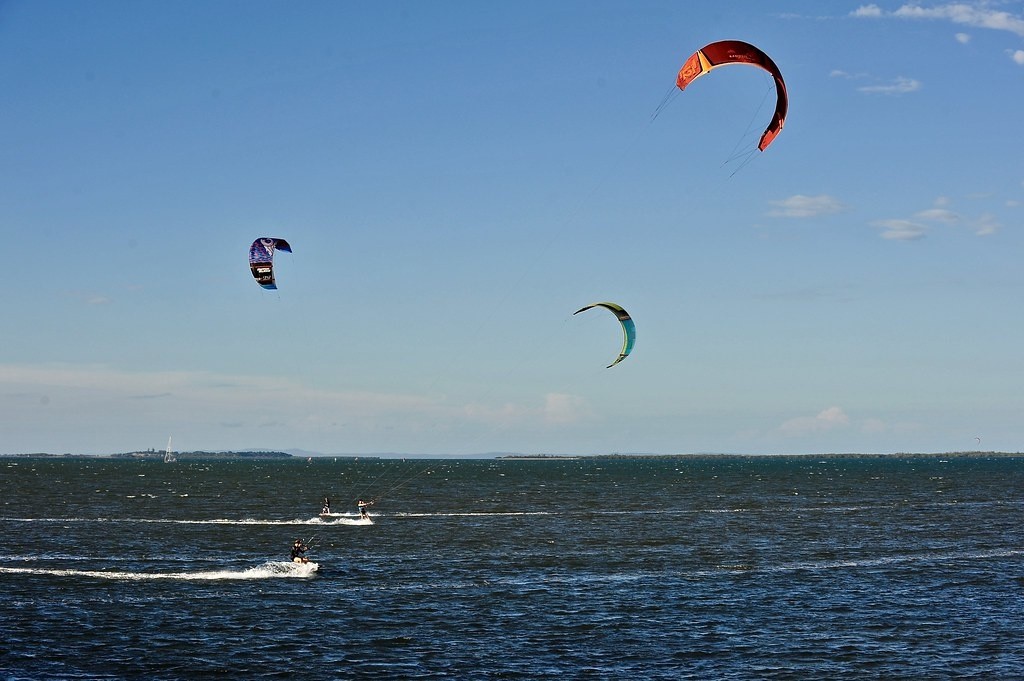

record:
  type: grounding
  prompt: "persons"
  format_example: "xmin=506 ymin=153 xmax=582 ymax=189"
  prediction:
xmin=358 ymin=499 xmax=371 ymax=519
xmin=291 ymin=539 xmax=309 ymax=564
xmin=322 ymin=497 xmax=330 ymax=513
